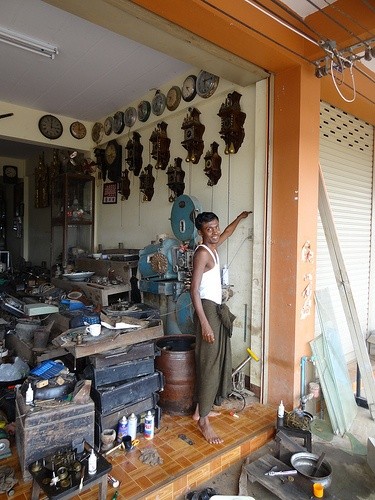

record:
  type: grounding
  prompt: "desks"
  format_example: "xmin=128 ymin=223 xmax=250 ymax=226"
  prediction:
xmin=27 ymin=441 xmax=113 ymax=500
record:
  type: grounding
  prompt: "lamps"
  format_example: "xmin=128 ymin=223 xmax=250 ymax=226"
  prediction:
xmin=0 ymin=26 xmax=59 ymax=60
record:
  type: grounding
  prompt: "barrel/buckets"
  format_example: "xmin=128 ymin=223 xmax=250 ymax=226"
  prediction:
xmin=154 ymin=334 xmax=198 ymax=418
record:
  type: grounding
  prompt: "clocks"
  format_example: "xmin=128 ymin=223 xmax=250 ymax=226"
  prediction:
xmin=38 ymin=114 xmax=63 ymax=140
xmin=92 ymin=69 xmax=247 ymax=203
xmin=69 ymin=121 xmax=87 ymax=139
xmin=2 ymin=165 xmax=18 ymax=179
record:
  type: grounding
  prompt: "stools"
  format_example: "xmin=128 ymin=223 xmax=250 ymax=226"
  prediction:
xmin=274 ymin=426 xmax=312 ymax=453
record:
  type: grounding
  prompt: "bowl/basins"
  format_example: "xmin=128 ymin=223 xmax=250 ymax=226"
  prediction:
xmin=93 ymin=254 xmax=101 ymax=258
xmin=290 ymin=451 xmax=333 ymax=489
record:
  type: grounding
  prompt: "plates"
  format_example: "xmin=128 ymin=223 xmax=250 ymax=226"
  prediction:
xmin=62 ymin=272 xmax=95 ymax=281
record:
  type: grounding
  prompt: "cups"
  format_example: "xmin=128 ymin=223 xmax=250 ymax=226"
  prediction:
xmin=86 ymin=324 xmax=101 ymax=337
xmin=313 ymin=482 xmax=323 ymax=498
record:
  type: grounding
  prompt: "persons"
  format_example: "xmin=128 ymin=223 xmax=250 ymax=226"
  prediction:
xmin=190 ymin=211 xmax=253 ymax=445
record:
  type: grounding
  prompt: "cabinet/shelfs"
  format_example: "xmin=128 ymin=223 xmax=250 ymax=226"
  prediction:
xmin=47 ymin=150 xmax=96 ymax=268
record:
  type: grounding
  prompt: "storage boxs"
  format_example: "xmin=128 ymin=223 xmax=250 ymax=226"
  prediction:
xmin=16 ymin=317 xmax=165 ymax=479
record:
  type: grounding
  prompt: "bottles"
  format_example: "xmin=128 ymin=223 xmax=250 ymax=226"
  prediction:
xmin=26 ymin=383 xmax=34 ymax=405
xmin=88 ymin=449 xmax=97 ymax=475
xmin=127 ymin=413 xmax=137 ymax=441
xmin=144 ymin=411 xmax=155 ymax=440
xmin=117 ymin=416 xmax=128 ymax=443
xmin=278 ymin=400 xmax=284 ymax=418
